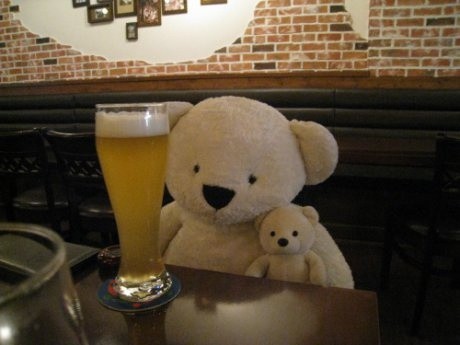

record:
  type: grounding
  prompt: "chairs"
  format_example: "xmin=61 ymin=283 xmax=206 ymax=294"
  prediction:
xmin=44 ymin=129 xmax=119 ymax=248
xmin=389 ymin=130 xmax=460 ymax=343
xmin=0 ymin=126 xmax=86 ymax=240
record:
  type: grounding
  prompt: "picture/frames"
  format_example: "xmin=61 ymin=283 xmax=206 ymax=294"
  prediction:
xmin=136 ymin=1 xmax=162 ymax=27
xmin=125 ymin=22 xmax=138 ymax=40
xmin=73 ymin=1 xmax=91 ymax=8
xmin=161 ymin=0 xmax=188 ymax=15
xmin=201 ymin=1 xmax=228 ymax=5
xmin=86 ymin=4 xmax=113 ymax=23
xmin=113 ymin=0 xmax=137 ymax=17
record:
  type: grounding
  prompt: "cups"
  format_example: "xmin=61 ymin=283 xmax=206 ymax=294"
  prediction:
xmin=94 ymin=102 xmax=174 ymax=303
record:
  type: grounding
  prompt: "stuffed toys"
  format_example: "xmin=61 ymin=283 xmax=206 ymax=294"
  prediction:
xmin=147 ymin=95 xmax=355 ymax=289
xmin=246 ymin=205 xmax=328 ymax=287
